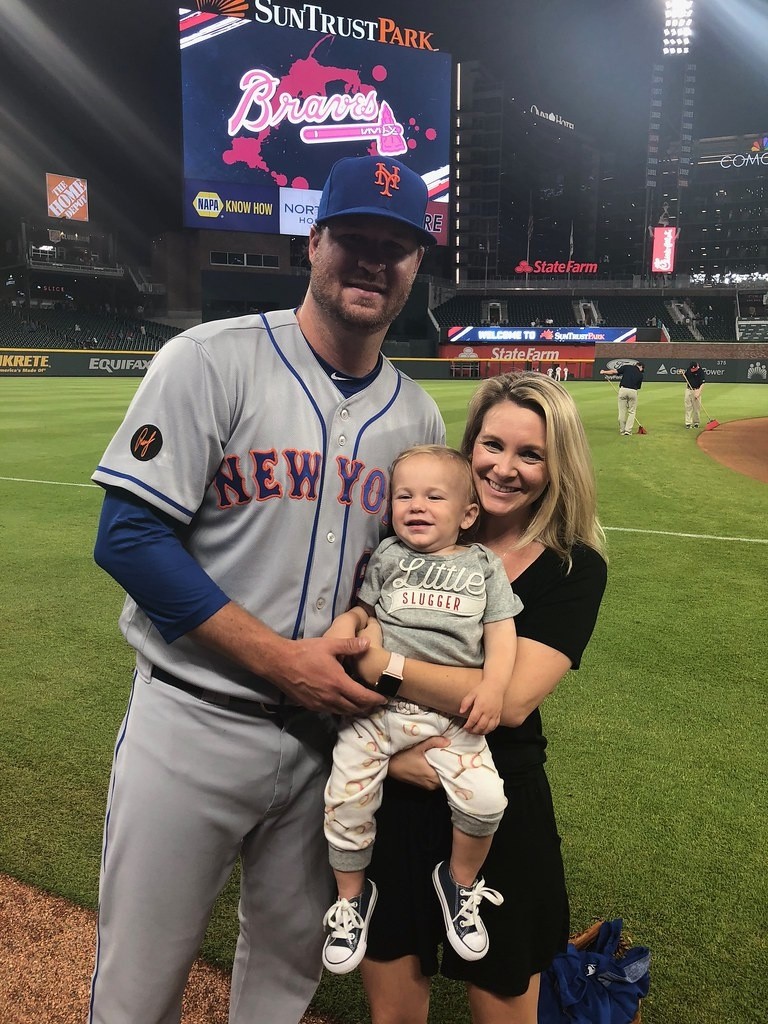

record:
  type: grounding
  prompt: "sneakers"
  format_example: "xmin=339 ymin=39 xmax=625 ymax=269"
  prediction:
xmin=320 ymin=877 xmax=380 ymax=976
xmin=432 ymin=861 xmax=491 ymax=961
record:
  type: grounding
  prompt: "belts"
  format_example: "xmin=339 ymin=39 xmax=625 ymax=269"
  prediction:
xmin=149 ymin=659 xmax=320 ymax=716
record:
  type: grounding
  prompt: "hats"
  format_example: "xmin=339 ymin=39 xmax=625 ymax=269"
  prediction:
xmin=689 ymin=360 xmax=697 ymax=369
xmin=638 ymin=362 xmax=645 ymax=372
xmin=315 ymin=154 xmax=442 ymax=242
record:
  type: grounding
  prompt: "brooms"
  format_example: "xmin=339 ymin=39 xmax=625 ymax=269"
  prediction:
xmin=678 ymin=368 xmax=720 ymax=431
xmin=600 ymin=372 xmax=647 ymax=437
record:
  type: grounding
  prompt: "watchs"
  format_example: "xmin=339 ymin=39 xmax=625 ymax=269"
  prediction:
xmin=374 ymin=651 xmax=407 ymax=699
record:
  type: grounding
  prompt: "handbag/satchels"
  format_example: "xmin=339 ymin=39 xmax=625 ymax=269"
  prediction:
xmin=536 ymin=920 xmax=652 ymax=1024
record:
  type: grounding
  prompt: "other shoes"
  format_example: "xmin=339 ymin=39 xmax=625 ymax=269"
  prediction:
xmin=625 ymin=431 xmax=632 ymax=435
xmin=694 ymin=425 xmax=699 ymax=428
xmin=620 ymin=432 xmax=625 ymax=436
xmin=685 ymin=425 xmax=691 ymax=428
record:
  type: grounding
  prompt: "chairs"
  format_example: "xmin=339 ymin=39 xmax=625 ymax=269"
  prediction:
xmin=0 ymin=305 xmax=185 ymax=351
xmin=432 ymin=294 xmax=768 ymax=342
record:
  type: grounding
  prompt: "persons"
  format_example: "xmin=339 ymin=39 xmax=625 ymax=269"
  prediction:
xmin=319 ymin=444 xmax=524 ymax=975
xmin=91 ymin=329 xmax=136 ymax=343
xmin=74 ymin=321 xmax=80 ymax=332
xmin=530 ymin=317 xmax=554 ymax=327
xmin=683 ymin=362 xmax=706 ymax=429
xmin=691 ymin=313 xmax=714 ymax=331
xmin=356 ymin=371 xmax=609 ymax=1024
xmin=90 ymin=157 xmax=448 ymax=1024
xmin=645 ymin=315 xmax=663 ymax=329
xmin=21 ymin=318 xmax=37 ymax=334
xmin=547 ymin=364 xmax=569 ymax=381
xmin=600 ymin=362 xmax=645 ymax=435
